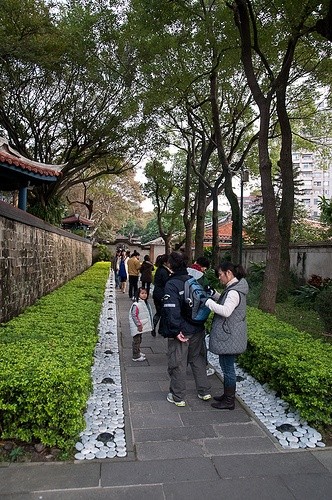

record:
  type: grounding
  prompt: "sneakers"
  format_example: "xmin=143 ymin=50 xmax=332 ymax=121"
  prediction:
xmin=198 ymin=394 xmax=211 ymax=400
xmin=205 ymin=368 xmax=215 ymax=376
xmin=132 ymin=356 xmax=146 ymax=362
xmin=139 ymin=352 xmax=145 ymax=356
xmin=166 ymin=393 xmax=186 ymax=406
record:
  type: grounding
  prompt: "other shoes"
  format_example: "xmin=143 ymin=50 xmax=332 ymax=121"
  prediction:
xmin=123 ymin=288 xmax=125 ymax=293
xmin=131 ymin=296 xmax=136 ymax=301
xmin=151 ymin=331 xmax=156 ymax=336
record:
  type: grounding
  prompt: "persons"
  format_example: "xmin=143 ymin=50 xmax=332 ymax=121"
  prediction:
xmin=200 ymin=262 xmax=248 ymax=410
xmin=114 ymin=247 xmax=174 ymax=337
xmin=158 ymin=251 xmax=211 ymax=407
xmin=128 ymin=287 xmax=154 ymax=362
xmin=187 ymin=256 xmax=215 ymax=377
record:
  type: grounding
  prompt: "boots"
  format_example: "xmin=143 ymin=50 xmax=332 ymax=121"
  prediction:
xmin=211 ymin=384 xmax=235 ymax=410
xmin=213 ymin=383 xmax=226 ymax=401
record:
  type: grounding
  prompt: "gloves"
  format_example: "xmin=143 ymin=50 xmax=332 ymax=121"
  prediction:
xmin=138 ymin=326 xmax=143 ymax=332
xmin=204 ymin=285 xmax=215 ymax=296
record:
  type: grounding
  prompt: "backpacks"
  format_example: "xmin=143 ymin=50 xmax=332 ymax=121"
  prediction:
xmin=170 ymin=275 xmax=215 ymax=326
